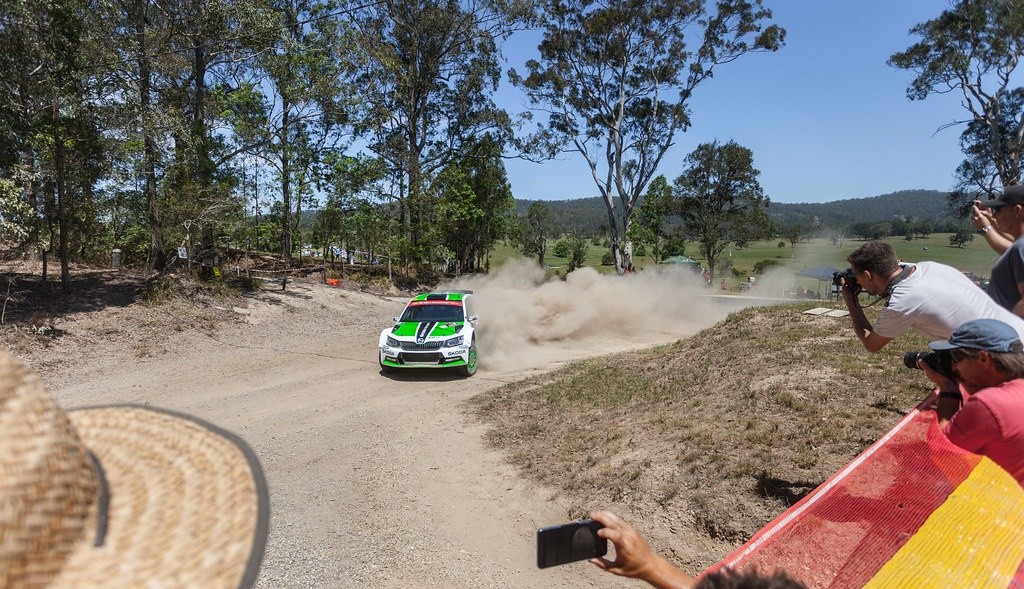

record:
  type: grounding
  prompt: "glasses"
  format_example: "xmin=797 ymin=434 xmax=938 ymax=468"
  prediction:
xmin=950 ymin=350 xmax=977 ymax=363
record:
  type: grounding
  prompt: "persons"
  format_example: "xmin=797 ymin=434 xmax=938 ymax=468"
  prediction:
xmin=840 ymin=242 xmax=1024 ymax=353
xmin=0 ymin=347 xmax=270 ymax=589
xmin=972 ymin=185 xmax=1024 ymax=319
xmin=588 ymin=510 xmax=810 ymax=589
xmin=721 ymin=278 xmax=728 ymax=290
xmin=624 ymin=266 xmax=644 ymax=278
xmin=335 ymin=248 xmax=340 ymax=260
xmin=703 ymin=268 xmax=710 ymax=283
xmin=918 ymin=318 xmax=1024 ymax=491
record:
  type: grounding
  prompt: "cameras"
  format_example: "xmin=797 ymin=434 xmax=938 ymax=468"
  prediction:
xmin=903 ymin=350 xmax=958 ymax=377
xmin=832 ymin=268 xmax=862 ymax=293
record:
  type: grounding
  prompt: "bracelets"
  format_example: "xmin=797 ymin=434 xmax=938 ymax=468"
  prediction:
xmin=939 ymin=392 xmax=961 ymax=400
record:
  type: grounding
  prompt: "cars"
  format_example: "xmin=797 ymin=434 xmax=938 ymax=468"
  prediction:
xmin=378 ymin=290 xmax=479 ymax=377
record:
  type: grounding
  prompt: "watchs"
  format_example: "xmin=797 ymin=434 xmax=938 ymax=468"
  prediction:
xmin=979 ymin=225 xmax=993 ymax=236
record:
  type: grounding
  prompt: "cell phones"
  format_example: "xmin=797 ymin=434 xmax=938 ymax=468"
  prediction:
xmin=537 ymin=520 xmax=607 ymax=569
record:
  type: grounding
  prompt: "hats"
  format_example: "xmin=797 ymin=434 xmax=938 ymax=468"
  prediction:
xmin=0 ymin=348 xmax=271 ymax=589
xmin=928 ymin=318 xmax=1021 ymax=353
xmin=981 ymin=186 xmax=1024 ymax=207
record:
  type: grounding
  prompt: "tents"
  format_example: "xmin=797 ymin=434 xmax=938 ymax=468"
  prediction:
xmin=790 ymin=266 xmax=842 ymax=299
xmin=658 ymin=255 xmax=700 ymax=271
